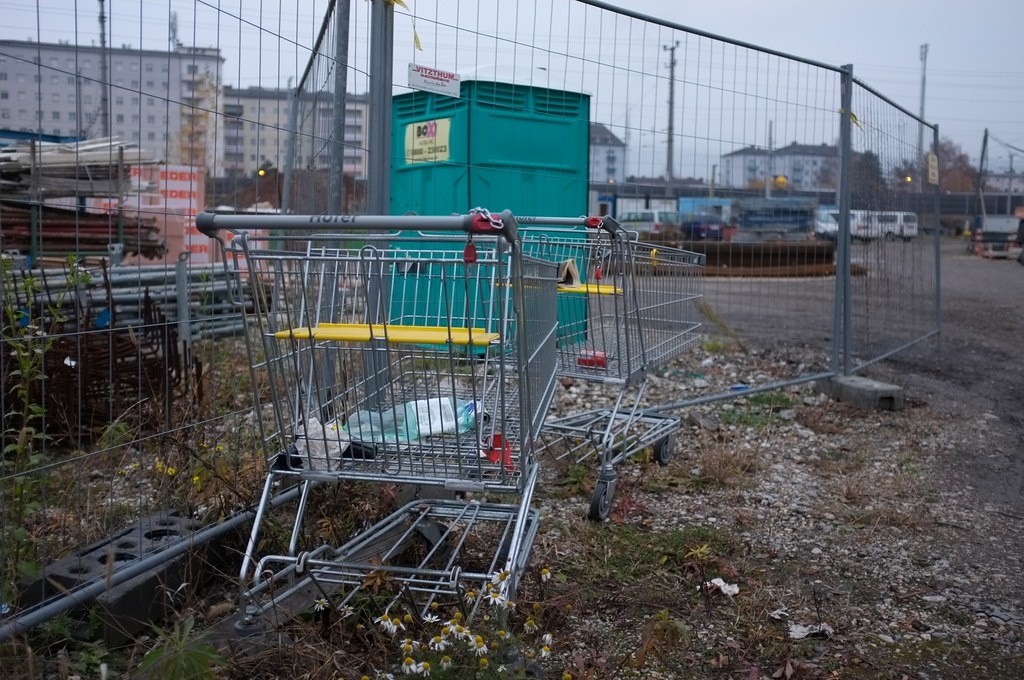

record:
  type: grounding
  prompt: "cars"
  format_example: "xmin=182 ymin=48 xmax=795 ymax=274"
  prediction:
xmin=681 ymin=215 xmax=723 ymax=241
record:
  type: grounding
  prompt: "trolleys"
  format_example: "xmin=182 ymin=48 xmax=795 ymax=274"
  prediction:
xmin=411 ymin=205 xmax=700 ymax=525
xmin=181 ymin=199 xmax=562 ymax=630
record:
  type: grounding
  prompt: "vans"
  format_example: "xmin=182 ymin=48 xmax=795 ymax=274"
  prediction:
xmin=877 ymin=211 xmax=919 ymax=243
xmin=616 ymin=209 xmax=680 ymax=235
xmin=816 ymin=208 xmax=878 ymax=243
